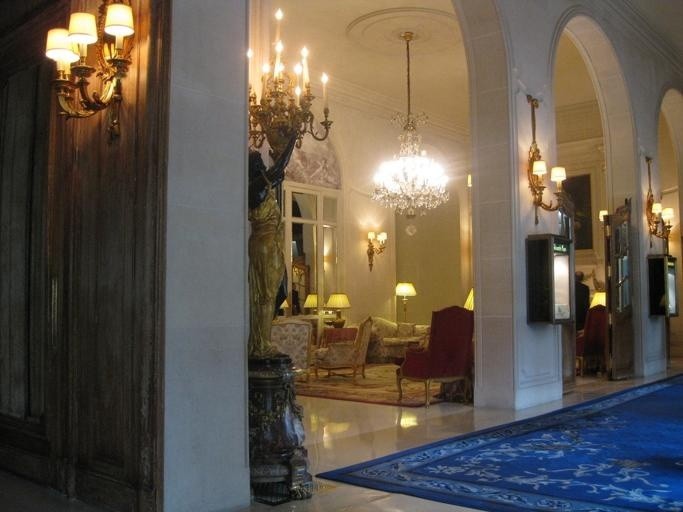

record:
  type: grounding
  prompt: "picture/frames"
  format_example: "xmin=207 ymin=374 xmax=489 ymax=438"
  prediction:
xmin=561 ymin=167 xmax=603 ymax=266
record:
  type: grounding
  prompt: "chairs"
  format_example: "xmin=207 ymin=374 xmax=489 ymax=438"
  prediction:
xmin=268 ymin=315 xmax=373 ymax=381
xmin=394 ymin=305 xmax=475 ymax=407
xmin=576 ymin=304 xmax=609 ymax=375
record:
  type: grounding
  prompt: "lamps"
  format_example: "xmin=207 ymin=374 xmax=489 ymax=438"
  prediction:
xmin=43 ymin=3 xmax=136 ymax=139
xmin=324 ymin=293 xmax=352 ymax=329
xmin=302 ymin=294 xmax=317 ymax=314
xmin=644 ymin=154 xmax=676 ymax=256
xmin=391 ymin=282 xmax=416 ymax=323
xmin=522 ymin=92 xmax=567 ymax=224
xmin=367 ymin=231 xmax=388 ymax=274
xmin=368 ymin=29 xmax=455 ymax=222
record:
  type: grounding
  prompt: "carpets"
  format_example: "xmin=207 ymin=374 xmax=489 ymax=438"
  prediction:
xmin=290 ymin=363 xmax=445 ymax=405
xmin=314 ymin=366 xmax=682 ymax=512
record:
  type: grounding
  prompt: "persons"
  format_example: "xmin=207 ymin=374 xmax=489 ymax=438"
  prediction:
xmin=574 ymin=270 xmax=592 ymax=331
xmin=247 ymin=129 xmax=299 ymax=364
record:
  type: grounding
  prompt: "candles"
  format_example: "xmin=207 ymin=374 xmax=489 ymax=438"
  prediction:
xmin=247 ymin=6 xmax=335 ymax=113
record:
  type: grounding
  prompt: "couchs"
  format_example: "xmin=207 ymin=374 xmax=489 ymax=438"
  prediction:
xmin=372 ymin=318 xmax=430 ymax=362
